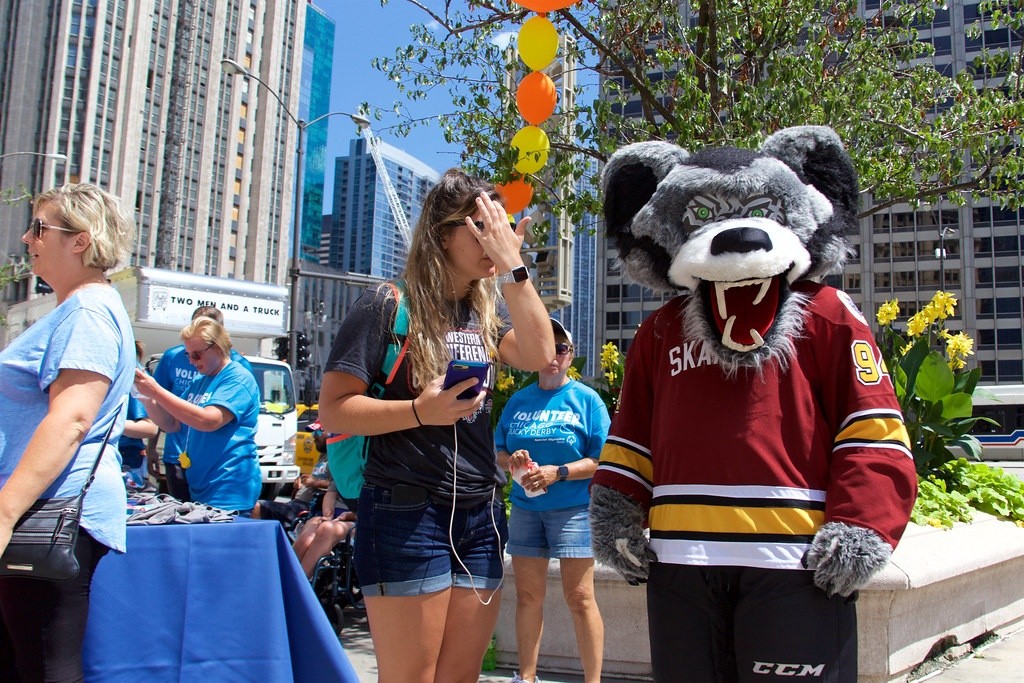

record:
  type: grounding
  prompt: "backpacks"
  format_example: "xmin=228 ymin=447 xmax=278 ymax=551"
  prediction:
xmin=324 ymin=280 xmax=412 ymax=513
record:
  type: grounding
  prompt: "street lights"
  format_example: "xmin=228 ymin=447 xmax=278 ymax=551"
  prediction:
xmin=221 ymin=59 xmax=371 ymax=403
xmin=922 ymin=195 xmax=955 ymax=358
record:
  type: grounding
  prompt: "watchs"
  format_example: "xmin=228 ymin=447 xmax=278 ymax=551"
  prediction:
xmin=497 ymin=265 xmax=529 ymax=285
xmin=556 ymin=465 xmax=569 ymax=482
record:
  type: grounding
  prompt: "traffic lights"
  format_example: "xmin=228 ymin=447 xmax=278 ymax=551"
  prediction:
xmin=274 ymin=338 xmax=289 ymax=360
xmin=297 ymin=333 xmax=311 ymax=370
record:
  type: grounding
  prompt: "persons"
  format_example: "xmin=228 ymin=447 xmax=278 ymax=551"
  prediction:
xmin=318 ymin=168 xmax=556 ymax=683
xmin=118 ymin=306 xmax=365 ymax=608
xmin=0 ymin=183 xmax=138 ymax=683
xmin=493 ymin=318 xmax=612 ymax=683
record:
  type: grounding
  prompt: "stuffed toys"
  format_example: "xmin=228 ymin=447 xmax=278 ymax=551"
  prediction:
xmin=588 ymin=126 xmax=918 ymax=683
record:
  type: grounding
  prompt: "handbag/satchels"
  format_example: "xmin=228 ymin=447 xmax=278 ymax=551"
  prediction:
xmin=1 ymin=486 xmax=84 ymax=588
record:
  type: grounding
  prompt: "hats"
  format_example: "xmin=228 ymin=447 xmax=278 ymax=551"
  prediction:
xmin=549 ymin=316 xmax=574 ymax=344
xmin=305 ymin=418 xmax=323 ymax=432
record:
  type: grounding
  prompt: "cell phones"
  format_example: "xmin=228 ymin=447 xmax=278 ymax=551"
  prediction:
xmin=442 ymin=360 xmax=488 ymax=400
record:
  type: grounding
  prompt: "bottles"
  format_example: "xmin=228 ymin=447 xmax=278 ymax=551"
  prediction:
xmin=481 ymin=630 xmax=497 ymax=672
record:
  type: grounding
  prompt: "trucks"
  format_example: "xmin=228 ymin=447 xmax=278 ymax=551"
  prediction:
xmin=142 ymin=352 xmax=300 ymax=501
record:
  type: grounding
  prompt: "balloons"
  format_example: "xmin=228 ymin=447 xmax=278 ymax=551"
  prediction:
xmin=493 ymin=0 xmax=581 ymax=223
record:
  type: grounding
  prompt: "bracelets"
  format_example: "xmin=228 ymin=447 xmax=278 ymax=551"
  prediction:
xmin=412 ymin=399 xmax=424 ymax=427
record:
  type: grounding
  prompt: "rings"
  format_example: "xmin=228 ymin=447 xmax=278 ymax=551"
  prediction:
xmin=533 ymin=481 xmax=540 ymax=488
xmin=517 ymin=452 xmax=521 ymax=457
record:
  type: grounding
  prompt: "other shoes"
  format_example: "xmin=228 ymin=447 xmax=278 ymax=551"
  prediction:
xmin=510 ymin=671 xmax=539 ymax=683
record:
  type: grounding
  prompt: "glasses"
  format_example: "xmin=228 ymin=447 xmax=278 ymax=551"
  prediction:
xmin=309 ymin=430 xmax=323 ymax=436
xmin=28 ymin=217 xmax=83 ymax=240
xmin=185 ymin=341 xmax=218 ymax=361
xmin=451 ymin=220 xmax=517 ymax=245
xmin=553 ymin=343 xmax=572 ymax=356
